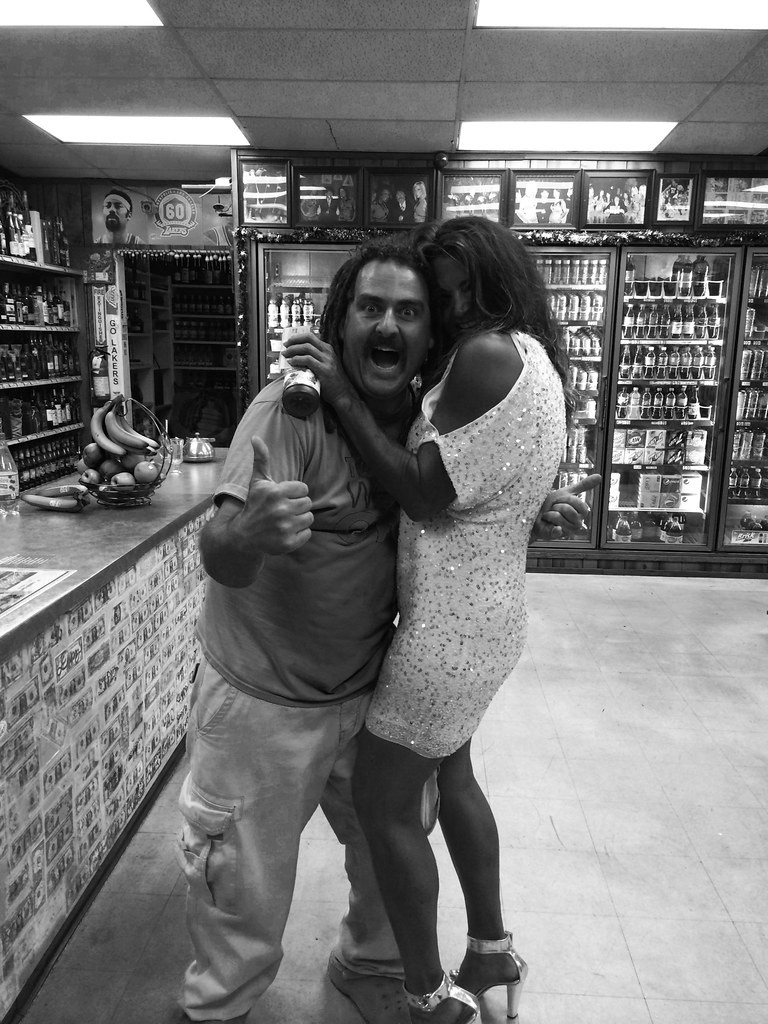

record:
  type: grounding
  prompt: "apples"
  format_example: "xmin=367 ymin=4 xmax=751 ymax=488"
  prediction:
xmin=77 ymin=443 xmax=160 ymax=493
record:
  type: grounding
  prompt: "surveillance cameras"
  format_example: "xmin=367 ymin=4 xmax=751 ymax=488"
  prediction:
xmin=140 ymin=201 xmax=155 ymax=214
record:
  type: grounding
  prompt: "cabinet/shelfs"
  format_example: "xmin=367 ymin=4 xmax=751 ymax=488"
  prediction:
xmin=0 ymin=209 xmax=93 ymax=493
xmin=124 ymin=254 xmax=174 ymax=436
xmin=172 ymin=282 xmax=237 ymax=371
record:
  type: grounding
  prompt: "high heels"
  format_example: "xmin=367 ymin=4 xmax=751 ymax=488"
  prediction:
xmin=450 ymin=931 xmax=528 ymax=1019
xmin=403 ymin=970 xmax=481 ymax=1024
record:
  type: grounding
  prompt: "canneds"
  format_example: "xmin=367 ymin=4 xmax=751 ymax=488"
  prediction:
xmin=729 ymin=264 xmax=768 ymax=462
xmin=543 ymin=293 xmax=603 ymax=503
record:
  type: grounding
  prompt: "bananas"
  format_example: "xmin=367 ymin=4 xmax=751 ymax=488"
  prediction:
xmin=89 ymin=394 xmax=161 ymax=456
xmin=20 ymin=484 xmax=91 ymax=513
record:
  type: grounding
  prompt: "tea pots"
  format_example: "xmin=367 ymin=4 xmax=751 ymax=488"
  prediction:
xmin=175 ymin=432 xmax=216 ymax=462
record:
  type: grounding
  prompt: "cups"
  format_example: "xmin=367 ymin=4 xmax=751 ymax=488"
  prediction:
xmin=170 ymin=438 xmax=184 ymax=464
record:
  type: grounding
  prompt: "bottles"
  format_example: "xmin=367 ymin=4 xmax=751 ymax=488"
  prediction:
xmin=525 ymin=253 xmax=768 ymax=546
xmin=266 ymin=291 xmax=421 ymax=419
xmin=123 ymin=253 xmax=237 ymax=447
xmin=0 ymin=184 xmax=81 ymax=511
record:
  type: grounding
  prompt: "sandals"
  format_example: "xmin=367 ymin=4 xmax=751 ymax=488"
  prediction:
xmin=328 ymin=949 xmax=412 ymax=1024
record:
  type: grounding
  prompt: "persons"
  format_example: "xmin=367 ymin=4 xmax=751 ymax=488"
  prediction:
xmin=177 ymin=234 xmax=603 ymax=1024
xmin=280 ymin=218 xmax=577 ymax=1024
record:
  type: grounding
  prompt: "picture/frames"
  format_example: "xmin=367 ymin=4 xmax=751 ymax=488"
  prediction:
xmin=236 ymin=155 xmax=293 ymax=228
xmin=508 ymin=166 xmax=579 ymax=232
xmin=696 ymin=169 xmax=768 ymax=233
xmin=437 ymin=164 xmax=507 ymax=231
xmin=363 ymin=162 xmax=436 ymax=230
xmin=578 ymin=170 xmax=655 ymax=230
xmin=291 ymin=165 xmax=364 ymax=228
xmin=652 ymin=171 xmax=696 ymax=225
